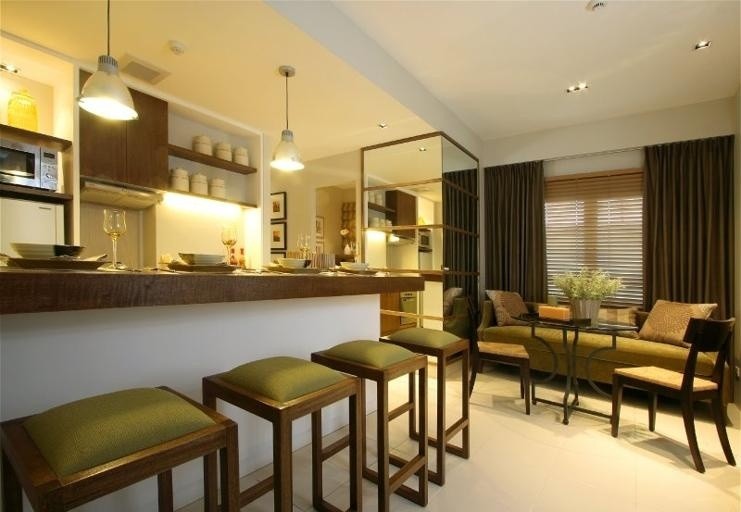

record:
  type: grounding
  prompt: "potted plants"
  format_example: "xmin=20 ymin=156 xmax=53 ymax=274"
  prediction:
xmin=552 ymin=265 xmax=626 ymax=328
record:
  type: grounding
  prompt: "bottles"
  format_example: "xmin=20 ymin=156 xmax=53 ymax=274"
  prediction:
xmin=170 ymin=133 xmax=249 ymax=197
xmin=230 ymin=248 xmax=246 ymax=271
xmin=7 ymin=88 xmax=38 ymax=133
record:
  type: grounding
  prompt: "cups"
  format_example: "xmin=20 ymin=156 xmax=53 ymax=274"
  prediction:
xmin=367 ymin=190 xmax=383 ymax=207
xmin=157 ymin=256 xmax=170 ymax=271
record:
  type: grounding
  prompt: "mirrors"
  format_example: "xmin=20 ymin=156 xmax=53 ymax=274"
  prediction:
xmin=361 ymin=131 xmax=481 ymax=368
xmin=316 ymin=180 xmax=356 ymax=268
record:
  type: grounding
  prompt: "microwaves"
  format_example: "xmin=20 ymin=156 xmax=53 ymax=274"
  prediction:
xmin=418 ymin=232 xmax=432 ymax=250
xmin=0 ymin=138 xmax=58 ymax=192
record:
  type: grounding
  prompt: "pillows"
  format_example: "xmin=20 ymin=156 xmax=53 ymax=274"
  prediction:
xmin=638 ymin=299 xmax=718 ymax=349
xmin=586 ymin=306 xmax=639 ymax=340
xmin=485 ymin=290 xmax=531 ymax=326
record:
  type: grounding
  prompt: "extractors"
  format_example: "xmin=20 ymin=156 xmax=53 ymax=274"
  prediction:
xmin=81 ymin=180 xmax=159 ymax=212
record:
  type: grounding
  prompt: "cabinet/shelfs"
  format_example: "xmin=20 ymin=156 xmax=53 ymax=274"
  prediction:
xmin=80 ymin=70 xmax=168 ymax=194
xmin=1 ymin=30 xmax=79 ymax=246
xmin=386 ymin=189 xmax=417 ymax=239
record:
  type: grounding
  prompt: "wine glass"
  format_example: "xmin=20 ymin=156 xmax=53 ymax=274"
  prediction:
xmin=100 ymin=208 xmax=127 ymax=270
xmin=220 ymin=228 xmax=238 ymax=266
xmin=297 ymin=232 xmax=313 ymax=270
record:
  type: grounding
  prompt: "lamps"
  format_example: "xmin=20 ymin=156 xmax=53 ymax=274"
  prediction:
xmin=270 ymin=66 xmax=306 ymax=171
xmin=75 ymin=1 xmax=139 ymax=121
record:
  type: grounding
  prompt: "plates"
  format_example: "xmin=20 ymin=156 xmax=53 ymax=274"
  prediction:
xmin=169 ymin=262 xmax=241 ymax=273
xmin=1 ymin=254 xmax=122 ymax=269
xmin=266 ymin=256 xmax=379 ymax=275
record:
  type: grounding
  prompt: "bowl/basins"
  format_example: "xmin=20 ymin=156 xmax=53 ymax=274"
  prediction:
xmin=179 ymin=250 xmax=229 ymax=264
xmin=369 ymin=217 xmax=393 ymax=233
xmin=11 ymin=243 xmax=86 ymax=258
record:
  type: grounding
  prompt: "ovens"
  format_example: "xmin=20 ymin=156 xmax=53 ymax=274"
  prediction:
xmin=399 ymin=292 xmax=417 ymax=325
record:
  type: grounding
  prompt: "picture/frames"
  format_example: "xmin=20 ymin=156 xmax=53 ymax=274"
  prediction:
xmin=316 ymin=216 xmax=324 ymax=254
xmin=271 ymin=192 xmax=287 ymax=263
